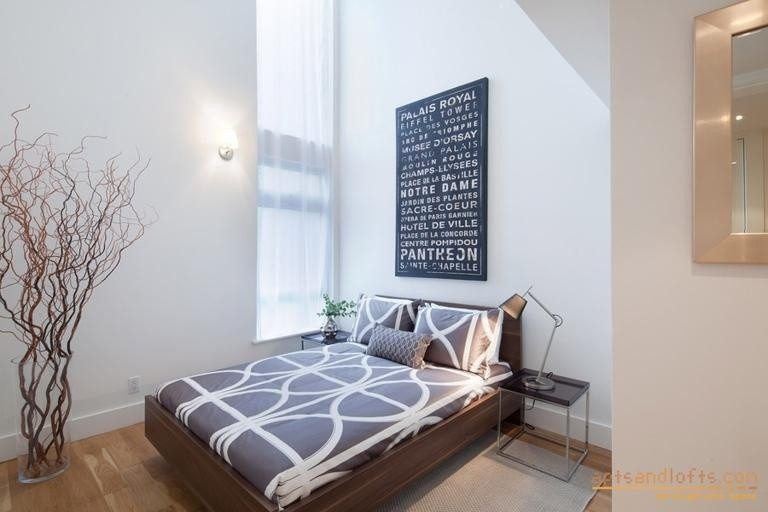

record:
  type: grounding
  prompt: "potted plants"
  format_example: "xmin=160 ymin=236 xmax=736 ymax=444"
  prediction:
xmin=316 ymin=293 xmax=358 ymax=339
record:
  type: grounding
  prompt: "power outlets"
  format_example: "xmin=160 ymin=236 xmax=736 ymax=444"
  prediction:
xmin=128 ymin=376 xmax=140 ymax=395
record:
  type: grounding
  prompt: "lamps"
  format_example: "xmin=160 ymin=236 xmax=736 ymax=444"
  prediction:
xmin=497 ymin=285 xmax=563 ymax=392
xmin=218 ymin=128 xmax=239 ymax=160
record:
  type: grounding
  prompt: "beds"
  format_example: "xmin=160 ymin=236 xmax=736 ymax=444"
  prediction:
xmin=146 ymin=296 xmax=525 ymax=512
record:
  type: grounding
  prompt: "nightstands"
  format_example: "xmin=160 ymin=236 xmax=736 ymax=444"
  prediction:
xmin=495 ymin=369 xmax=589 ymax=483
xmin=302 ymin=330 xmax=352 ymax=350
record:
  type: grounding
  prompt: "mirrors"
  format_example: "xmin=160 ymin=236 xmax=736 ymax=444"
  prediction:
xmin=692 ymin=0 xmax=768 ymax=263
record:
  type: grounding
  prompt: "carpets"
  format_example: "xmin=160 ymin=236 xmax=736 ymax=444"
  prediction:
xmin=373 ymin=430 xmax=608 ymax=512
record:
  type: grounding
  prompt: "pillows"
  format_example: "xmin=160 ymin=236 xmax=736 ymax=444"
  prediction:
xmin=348 ymin=294 xmax=504 ymax=380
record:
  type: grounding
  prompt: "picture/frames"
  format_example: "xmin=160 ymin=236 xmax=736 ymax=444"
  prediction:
xmin=396 ymin=76 xmax=489 ymax=281
